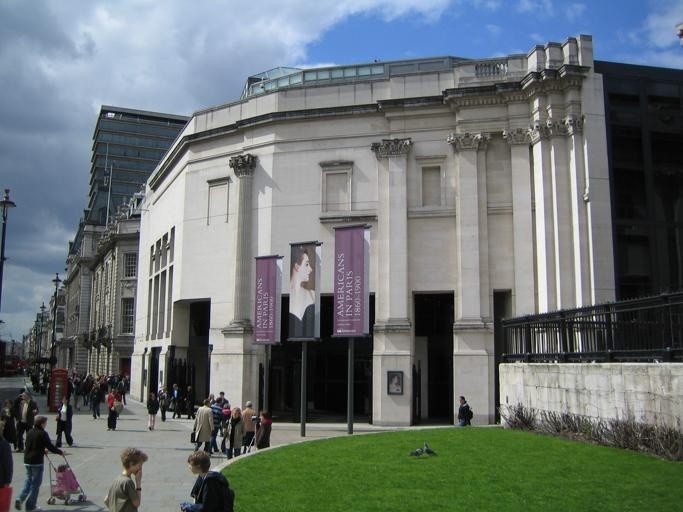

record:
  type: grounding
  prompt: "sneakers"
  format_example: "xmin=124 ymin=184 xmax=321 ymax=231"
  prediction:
xmin=16 ymin=498 xmax=21 ymax=511
xmin=27 ymin=506 xmax=41 ymax=512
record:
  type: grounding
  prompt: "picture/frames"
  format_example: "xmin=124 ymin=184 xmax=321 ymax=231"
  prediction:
xmin=385 ymin=369 xmax=404 ymax=396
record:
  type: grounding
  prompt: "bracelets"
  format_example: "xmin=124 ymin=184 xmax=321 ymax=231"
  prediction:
xmin=135 ymin=487 xmax=142 ymax=491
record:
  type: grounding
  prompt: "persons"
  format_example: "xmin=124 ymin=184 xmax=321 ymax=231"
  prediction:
xmin=0 ymin=421 xmax=13 ymax=489
xmin=15 ymin=415 xmax=64 ymax=512
xmin=457 ymin=396 xmax=470 ymax=426
xmin=103 ymin=447 xmax=149 ymax=511
xmin=289 ymin=245 xmax=315 ymax=338
xmin=388 ymin=372 xmax=402 ymax=394
xmin=179 ymin=449 xmax=235 ymax=511
xmin=0 ymin=354 xmax=273 ymax=470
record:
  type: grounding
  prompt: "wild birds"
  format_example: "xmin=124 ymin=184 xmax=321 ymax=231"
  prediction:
xmin=423 ymin=441 xmax=437 ymax=457
xmin=411 ymin=447 xmax=423 ymax=457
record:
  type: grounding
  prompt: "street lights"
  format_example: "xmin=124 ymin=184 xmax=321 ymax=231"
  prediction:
xmin=0 ymin=189 xmax=16 ymax=305
xmin=50 ymin=270 xmax=61 ymax=377
xmin=22 ymin=301 xmax=46 ymax=372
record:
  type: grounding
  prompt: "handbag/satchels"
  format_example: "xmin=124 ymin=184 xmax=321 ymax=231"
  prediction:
xmin=191 ymin=432 xmax=199 ymax=443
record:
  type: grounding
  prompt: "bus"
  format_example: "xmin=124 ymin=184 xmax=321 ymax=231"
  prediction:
xmin=4 ymin=355 xmax=19 ymax=376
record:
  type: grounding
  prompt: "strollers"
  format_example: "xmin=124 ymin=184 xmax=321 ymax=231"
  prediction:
xmin=45 ymin=449 xmax=89 ymax=507
